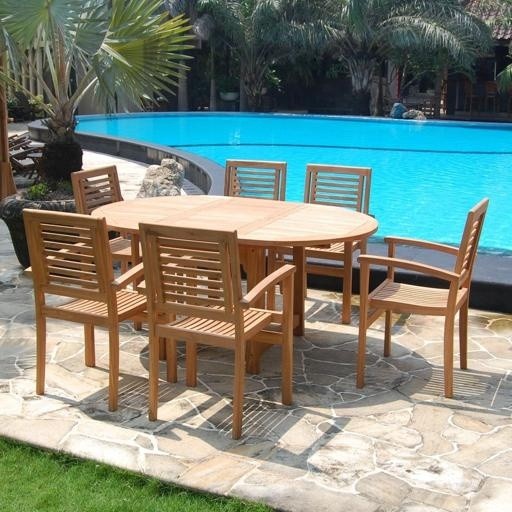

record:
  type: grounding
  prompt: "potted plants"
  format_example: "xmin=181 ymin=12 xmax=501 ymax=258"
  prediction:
xmin=1 ymin=1 xmax=198 ymax=272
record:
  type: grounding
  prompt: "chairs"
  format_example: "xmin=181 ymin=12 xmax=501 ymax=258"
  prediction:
xmin=9 ymin=132 xmax=45 ymax=185
xmin=211 ymin=160 xmax=288 ymax=312
xmin=71 ymin=164 xmax=179 ymax=333
xmin=136 ymin=222 xmax=297 ymax=441
xmin=356 ymin=197 xmax=491 ymax=398
xmin=266 ymin=162 xmax=372 ymax=326
xmin=21 ymin=207 xmax=147 ymax=413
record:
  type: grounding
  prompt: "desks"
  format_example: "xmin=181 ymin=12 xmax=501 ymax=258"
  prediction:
xmin=89 ymin=194 xmax=380 ymax=375
